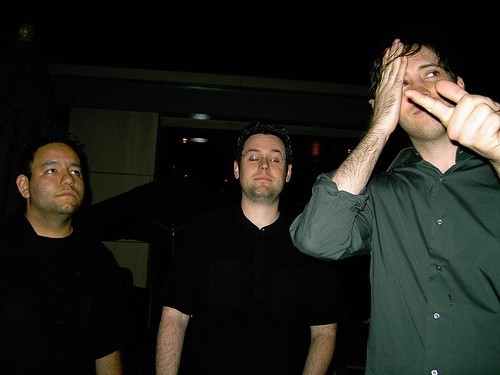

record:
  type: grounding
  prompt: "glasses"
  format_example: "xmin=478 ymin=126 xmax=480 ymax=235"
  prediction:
xmin=240 ymin=152 xmax=287 ymax=165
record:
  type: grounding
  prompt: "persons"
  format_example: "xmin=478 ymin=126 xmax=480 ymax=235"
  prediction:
xmin=154 ymin=122 xmax=344 ymax=375
xmin=289 ymin=33 xmax=500 ymax=375
xmin=0 ymin=135 xmax=132 ymax=375
